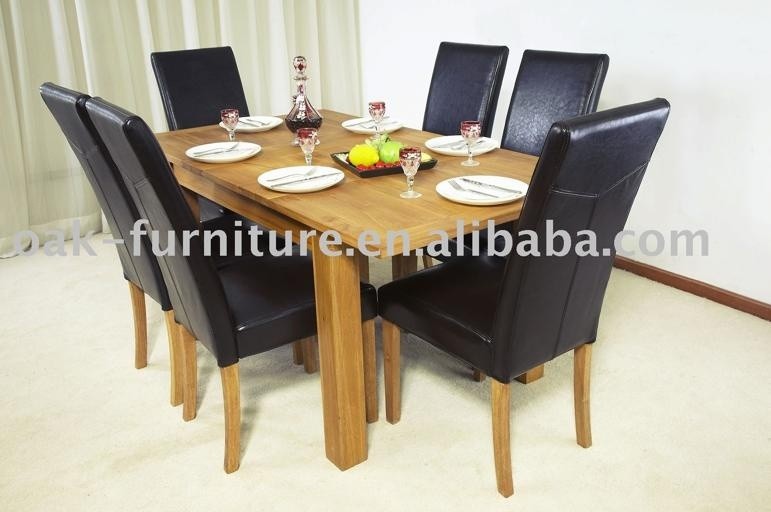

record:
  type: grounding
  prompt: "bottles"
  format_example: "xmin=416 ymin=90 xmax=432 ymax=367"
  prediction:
xmin=286 ymin=55 xmax=323 ymax=146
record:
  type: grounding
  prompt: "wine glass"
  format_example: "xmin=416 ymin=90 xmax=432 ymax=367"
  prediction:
xmin=296 ymin=128 xmax=317 ymax=165
xmin=368 ymin=102 xmax=387 ymax=140
xmin=221 ymin=109 xmax=239 ymax=141
xmin=460 ymin=120 xmax=482 ymax=168
xmin=398 ymin=147 xmax=423 ymax=200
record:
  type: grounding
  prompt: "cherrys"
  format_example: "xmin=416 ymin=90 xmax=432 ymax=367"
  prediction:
xmin=356 ymin=160 xmax=401 ymax=171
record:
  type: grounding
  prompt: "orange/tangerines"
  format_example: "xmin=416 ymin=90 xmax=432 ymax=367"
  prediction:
xmin=350 ymin=145 xmax=378 ymax=166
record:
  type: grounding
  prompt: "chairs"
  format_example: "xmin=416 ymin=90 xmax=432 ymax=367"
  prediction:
xmin=410 ymin=49 xmax=613 ymax=379
xmin=152 ymin=45 xmax=251 ymax=222
xmin=354 ymin=39 xmax=511 ymax=288
xmin=377 ymin=99 xmax=672 ymax=495
xmin=36 ymin=83 xmax=306 ymax=404
xmin=85 ymin=96 xmax=377 ymax=475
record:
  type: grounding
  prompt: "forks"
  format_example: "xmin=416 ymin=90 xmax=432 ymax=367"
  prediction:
xmin=450 ymin=180 xmax=496 ymax=199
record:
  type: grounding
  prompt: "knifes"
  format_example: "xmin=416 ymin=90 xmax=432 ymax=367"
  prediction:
xmin=464 ymin=178 xmax=522 ymax=194
xmin=271 ymin=173 xmax=343 ymax=187
xmin=433 ymin=140 xmax=463 ymax=148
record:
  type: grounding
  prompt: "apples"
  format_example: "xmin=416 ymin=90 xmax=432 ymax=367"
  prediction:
xmin=380 ymin=141 xmax=404 ymax=163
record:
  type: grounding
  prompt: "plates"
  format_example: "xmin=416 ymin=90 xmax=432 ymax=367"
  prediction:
xmin=258 ymin=165 xmax=345 ymax=193
xmin=437 ymin=176 xmax=529 ymax=205
xmin=343 ymin=118 xmax=404 ymax=135
xmin=220 ymin=117 xmax=284 ymax=133
xmin=425 ymin=134 xmax=498 ymax=157
xmin=186 ymin=142 xmax=263 ymax=164
